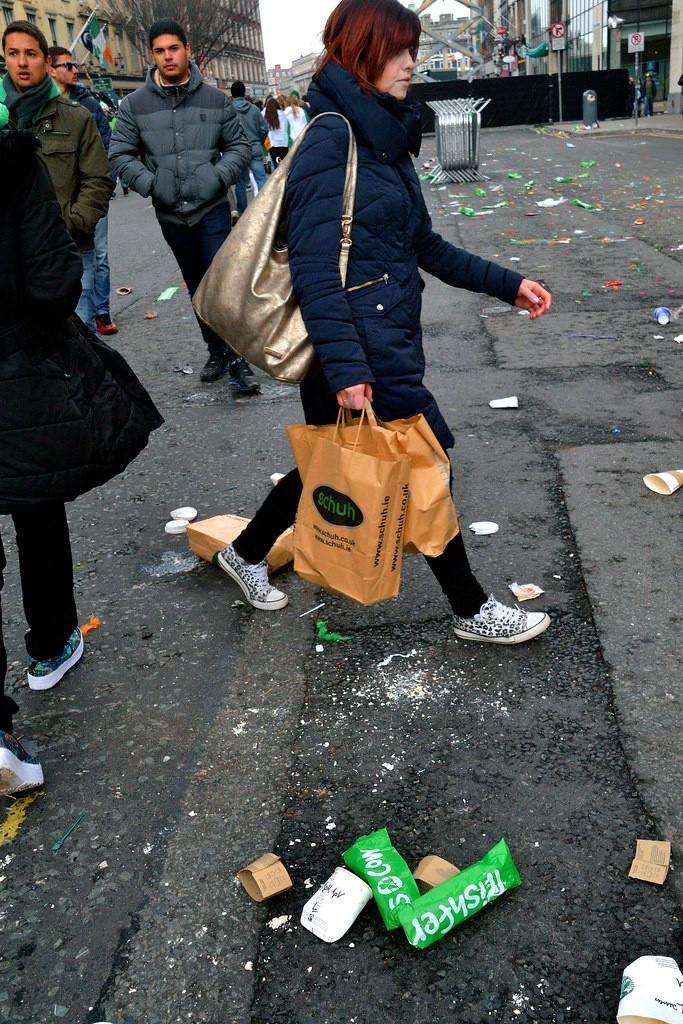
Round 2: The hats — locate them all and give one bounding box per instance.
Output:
[289,91,299,97]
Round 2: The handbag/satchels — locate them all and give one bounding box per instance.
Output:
[191,111,357,384]
[185,397,461,607]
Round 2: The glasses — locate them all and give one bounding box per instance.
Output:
[54,62,78,71]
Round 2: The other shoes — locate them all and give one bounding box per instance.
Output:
[110,192,116,199]
[231,210,239,226]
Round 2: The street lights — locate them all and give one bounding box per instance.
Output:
[114,53,125,98]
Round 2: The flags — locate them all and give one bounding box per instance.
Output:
[77,12,113,67]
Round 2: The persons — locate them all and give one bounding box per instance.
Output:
[0,103,165,795]
[629,77,642,118]
[0,20,311,392]
[217,0,552,645]
[678,73,683,117]
[642,72,656,119]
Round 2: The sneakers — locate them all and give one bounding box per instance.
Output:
[227,356,261,393]
[217,542,288,610]
[96,313,119,335]
[28,627,84,690]
[0,730,44,797]
[201,352,227,381]
[453,593,550,644]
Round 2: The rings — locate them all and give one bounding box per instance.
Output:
[539,298,542,300]
[343,400,348,404]
